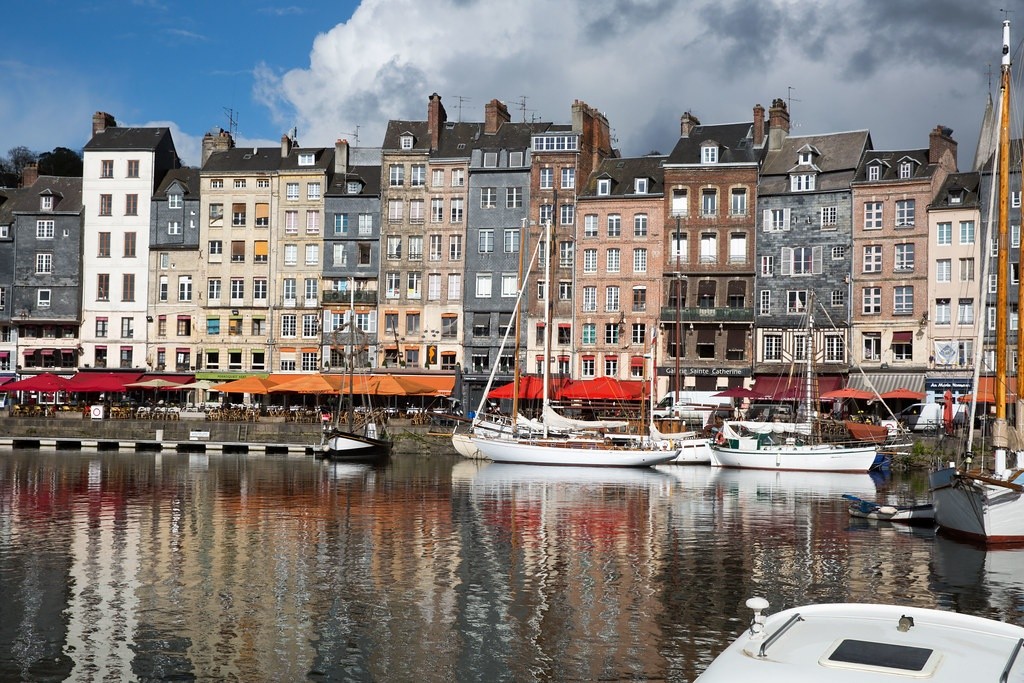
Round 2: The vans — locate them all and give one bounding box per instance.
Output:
[653,390,735,422]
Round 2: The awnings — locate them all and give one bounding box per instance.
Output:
[751,376,844,403]
[22,349,35,355]
[846,375,925,400]
[71,372,143,381]
[0,352,10,360]
[41,348,55,355]
[267,374,456,396]
[963,376,1018,403]
[61,349,73,353]
[891,332,912,344]
[137,375,196,391]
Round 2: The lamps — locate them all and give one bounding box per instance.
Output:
[232,310,239,315]
[146,316,153,322]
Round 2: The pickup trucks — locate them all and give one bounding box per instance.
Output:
[888,404,968,436]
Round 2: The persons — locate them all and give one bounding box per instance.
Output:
[224,401,231,409]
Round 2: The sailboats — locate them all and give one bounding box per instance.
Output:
[709,288,917,474]
[323,276,393,456]
[705,467,876,509]
[453,221,715,467]
[923,10,1024,545]
[450,460,692,598]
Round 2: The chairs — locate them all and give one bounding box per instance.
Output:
[13,402,432,426]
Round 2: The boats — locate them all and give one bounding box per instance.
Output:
[847,503,937,524]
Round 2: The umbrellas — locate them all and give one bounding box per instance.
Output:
[876,388,928,424]
[0,373,97,408]
[957,392,1017,422]
[121,373,437,416]
[821,388,875,412]
[488,375,563,418]
[709,387,765,421]
[556,375,653,415]
[770,387,821,404]
[944,390,953,435]
